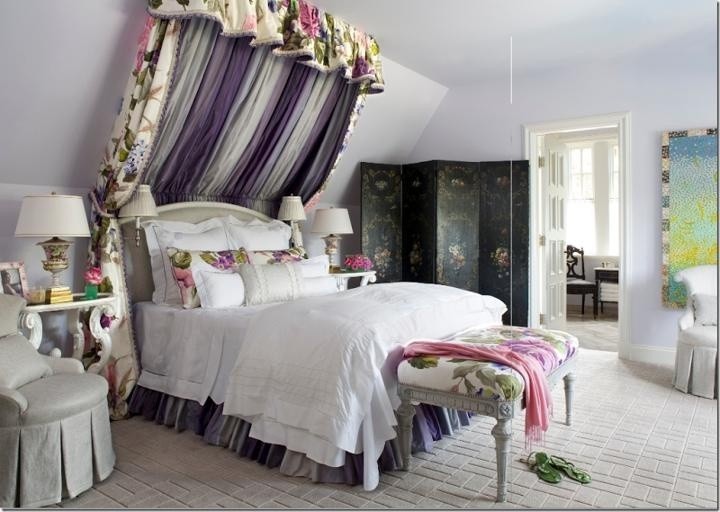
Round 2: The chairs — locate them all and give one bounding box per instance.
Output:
[1,287,114,510]
[671,262,718,401]
[563,243,597,319]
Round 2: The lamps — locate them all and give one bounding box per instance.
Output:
[118,181,155,249]
[309,203,353,272]
[277,193,308,250]
[15,191,94,299]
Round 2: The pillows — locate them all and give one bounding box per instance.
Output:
[691,289,718,328]
[132,215,343,312]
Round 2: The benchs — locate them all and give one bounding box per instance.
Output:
[391,322,582,501]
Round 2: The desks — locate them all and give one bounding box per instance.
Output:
[591,266,618,317]
[327,269,377,292]
[19,290,124,373]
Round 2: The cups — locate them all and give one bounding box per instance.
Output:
[29,290,46,305]
[602,262,610,268]
[83,285,98,299]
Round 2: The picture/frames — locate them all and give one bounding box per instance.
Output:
[1,259,29,301]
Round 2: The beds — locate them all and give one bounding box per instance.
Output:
[104,201,511,483]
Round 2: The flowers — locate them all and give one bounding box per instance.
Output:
[342,251,372,272]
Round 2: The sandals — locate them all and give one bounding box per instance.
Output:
[549,455,591,483]
[536,452,561,482]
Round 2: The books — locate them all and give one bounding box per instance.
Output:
[45,286,74,304]
